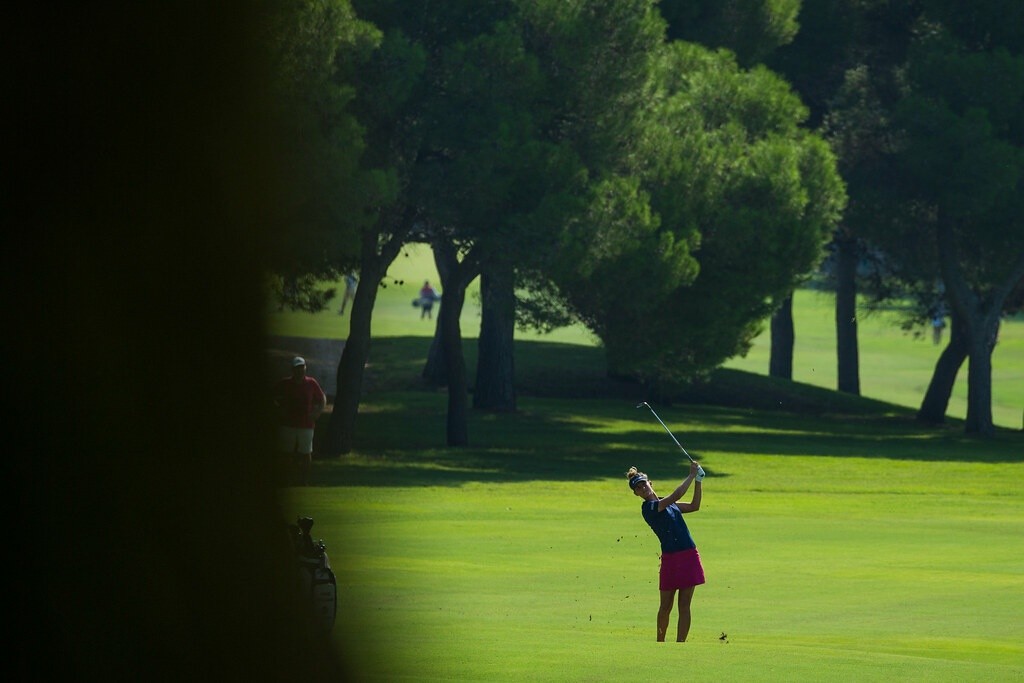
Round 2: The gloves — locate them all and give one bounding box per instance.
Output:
[695,466,705,482]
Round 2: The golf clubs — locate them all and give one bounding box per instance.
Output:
[637,402,704,477]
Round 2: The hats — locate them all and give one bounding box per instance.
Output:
[293,357,305,367]
[630,476,648,486]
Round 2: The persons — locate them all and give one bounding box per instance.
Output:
[419,282,442,319]
[626,460,705,642]
[271,357,326,463]
[336,274,357,315]
